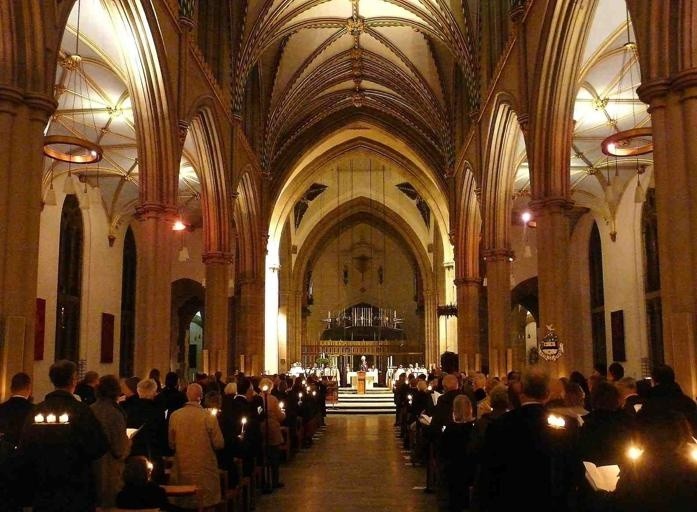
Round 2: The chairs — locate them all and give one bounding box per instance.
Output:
[95,416,320,512]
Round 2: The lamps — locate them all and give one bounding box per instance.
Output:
[602,4,655,202]
[41,0,103,209]
[176,223,195,262]
[524,220,536,258]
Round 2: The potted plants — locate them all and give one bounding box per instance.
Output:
[541,331,558,355]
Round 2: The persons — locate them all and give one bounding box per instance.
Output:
[392,363,697,512]
[361,361,369,372]
[0,358,327,512]
